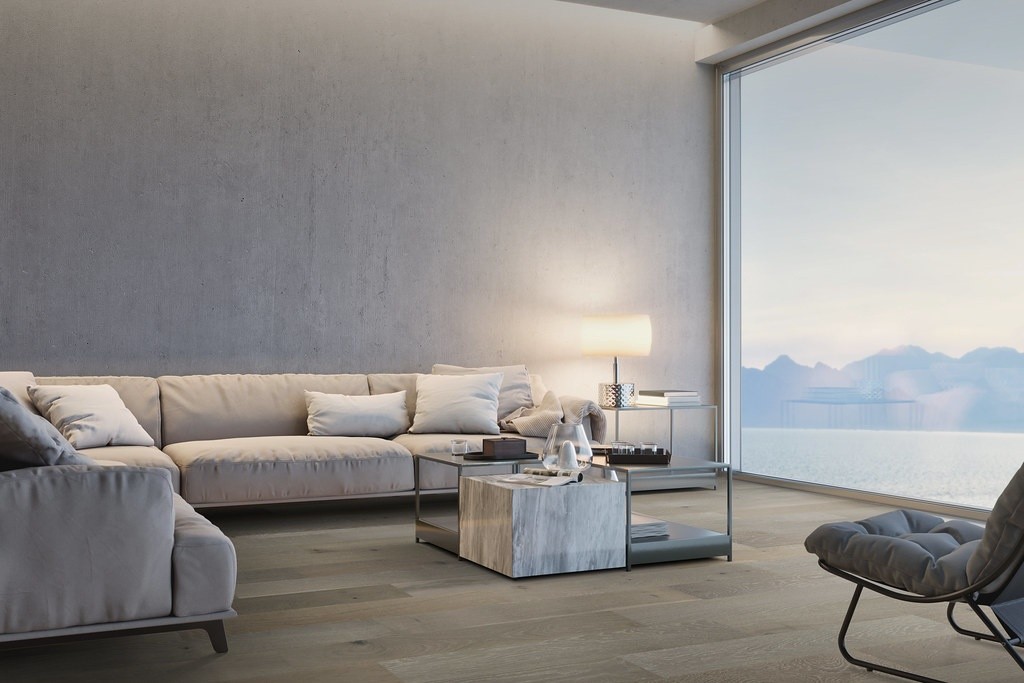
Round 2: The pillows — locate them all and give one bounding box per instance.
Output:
[0,386,97,465]
[407,373,502,435]
[302,388,410,437]
[26,385,155,450]
[431,362,530,421]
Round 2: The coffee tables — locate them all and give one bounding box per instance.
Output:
[413,453,540,560]
[578,454,733,571]
[458,473,628,578]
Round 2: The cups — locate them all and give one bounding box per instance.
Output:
[610,441,658,455]
[451,439,468,456]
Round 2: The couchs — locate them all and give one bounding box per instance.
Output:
[0,374,606,653]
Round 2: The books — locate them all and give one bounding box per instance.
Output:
[497,468,584,487]
[637,390,702,406]
[631,513,670,538]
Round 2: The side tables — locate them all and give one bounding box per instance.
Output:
[602,404,717,491]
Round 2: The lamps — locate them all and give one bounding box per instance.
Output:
[577,313,652,408]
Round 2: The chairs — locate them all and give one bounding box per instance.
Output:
[803,462,1024,683]
